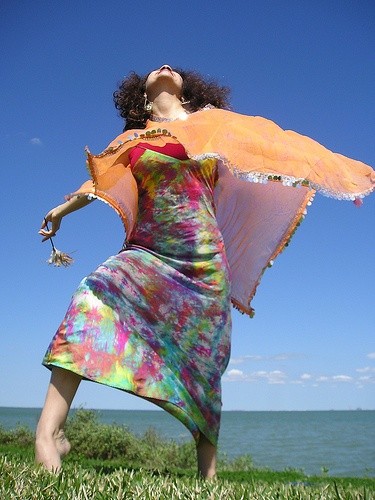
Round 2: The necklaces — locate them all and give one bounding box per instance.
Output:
[148,114,171,122]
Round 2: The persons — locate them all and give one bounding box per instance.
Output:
[33,63,374,489]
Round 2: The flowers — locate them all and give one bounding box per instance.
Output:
[43,217,76,269]
